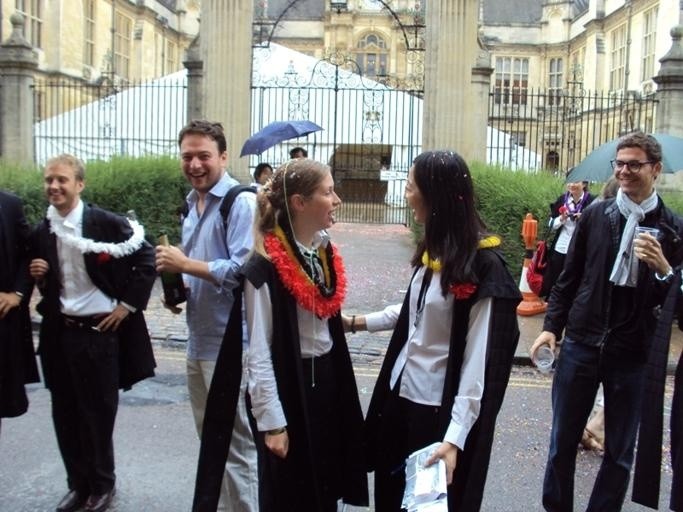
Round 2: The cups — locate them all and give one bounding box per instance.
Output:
[533,348,554,375]
[634,226,659,238]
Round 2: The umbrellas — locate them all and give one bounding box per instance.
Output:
[238,120,325,159]
[561,116,683,183]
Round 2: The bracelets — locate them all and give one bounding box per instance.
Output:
[266,429,284,434]
[16,291,22,296]
[351,315,356,334]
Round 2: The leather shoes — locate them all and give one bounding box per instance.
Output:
[85,485,116,510]
[56,487,85,512]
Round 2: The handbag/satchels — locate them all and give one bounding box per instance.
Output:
[527,240,553,297]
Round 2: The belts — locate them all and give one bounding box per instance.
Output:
[61,315,105,326]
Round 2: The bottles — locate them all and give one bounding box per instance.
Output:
[158,231,186,306]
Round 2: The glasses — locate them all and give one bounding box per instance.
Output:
[610,159,654,173]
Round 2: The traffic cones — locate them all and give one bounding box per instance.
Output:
[515,249,545,316]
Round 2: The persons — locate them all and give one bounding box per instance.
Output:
[538,170,593,342]
[250,163,274,192]
[155,119,259,512]
[189,156,372,512]
[290,147,306,161]
[630,261,683,512]
[0,189,41,420]
[532,132,683,512]
[339,151,524,512]
[23,153,157,512]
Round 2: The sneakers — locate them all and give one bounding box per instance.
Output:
[578,428,604,451]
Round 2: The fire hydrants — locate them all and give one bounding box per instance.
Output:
[521,214,538,246]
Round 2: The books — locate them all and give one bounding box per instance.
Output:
[402,442,448,512]
